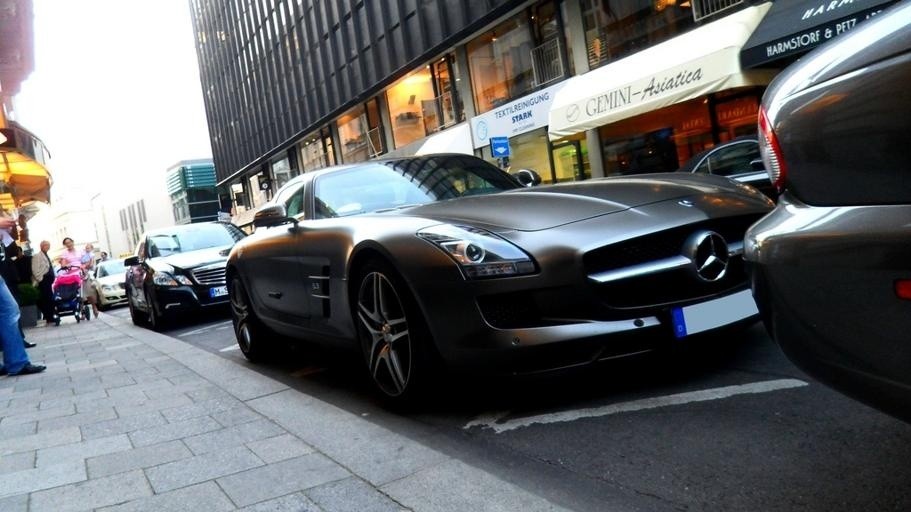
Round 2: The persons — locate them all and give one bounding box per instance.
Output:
[18,319,37,348]
[52,237,113,318]
[0,203,47,377]
[32,241,61,323]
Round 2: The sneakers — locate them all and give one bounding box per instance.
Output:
[80,313,85,320]
[94,311,98,318]
[0,363,46,376]
[43,316,56,322]
[22,339,36,348]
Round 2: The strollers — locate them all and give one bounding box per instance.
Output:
[51,266,90,326]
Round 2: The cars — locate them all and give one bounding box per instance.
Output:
[89,221,249,330]
